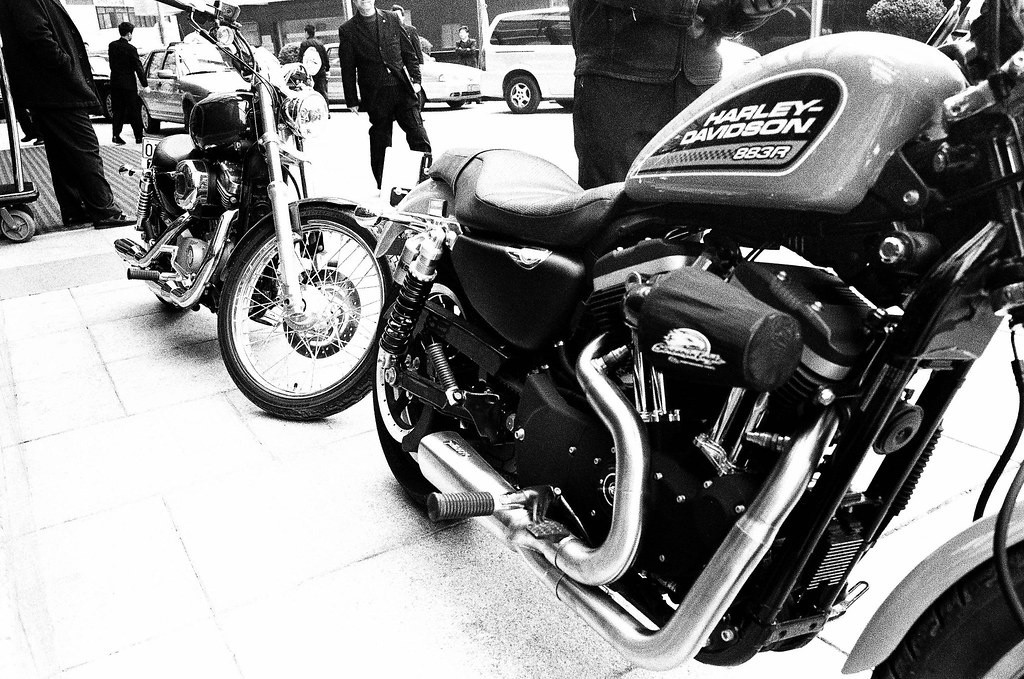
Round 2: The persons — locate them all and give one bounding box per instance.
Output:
[108,22,148,145]
[298,24,331,119]
[0,0,138,228]
[338,0,431,189]
[390,4,425,122]
[455,26,483,104]
[567,0,791,190]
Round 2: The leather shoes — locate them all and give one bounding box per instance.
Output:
[21,136,37,142]
[136,136,145,143]
[93,210,137,229]
[62,215,93,227]
[467,101,471,104]
[34,140,45,145]
[476,102,482,104]
[112,136,126,144]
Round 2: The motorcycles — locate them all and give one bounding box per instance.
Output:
[112,0,403,422]
[350,0,1023,679]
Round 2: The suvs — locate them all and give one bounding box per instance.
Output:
[482,7,764,116]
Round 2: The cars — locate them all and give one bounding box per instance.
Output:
[83,32,482,136]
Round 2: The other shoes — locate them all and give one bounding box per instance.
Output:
[329,114,331,119]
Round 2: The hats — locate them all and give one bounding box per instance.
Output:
[304,25,315,33]
